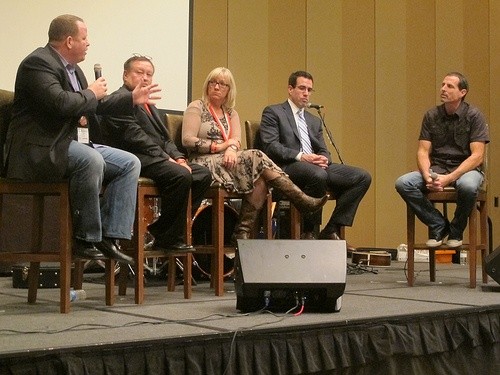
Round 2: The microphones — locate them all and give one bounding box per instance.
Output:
[307,103,324,108]
[95,64,104,103]
[431,173,437,180]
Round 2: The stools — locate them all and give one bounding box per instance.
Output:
[406,123,490,290]
[0,89,347,314]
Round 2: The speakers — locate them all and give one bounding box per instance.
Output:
[234,239,347,313]
[484,245,500,285]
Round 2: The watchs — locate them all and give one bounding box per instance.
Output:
[229,145,238,151]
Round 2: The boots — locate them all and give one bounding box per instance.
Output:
[267,172,329,217]
[231,198,261,246]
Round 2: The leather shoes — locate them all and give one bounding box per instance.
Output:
[304,232,317,240]
[72,238,104,259]
[94,236,133,263]
[318,231,357,251]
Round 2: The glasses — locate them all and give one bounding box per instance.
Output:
[132,52,152,60]
[209,80,229,87]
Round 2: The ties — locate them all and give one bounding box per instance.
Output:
[297,110,312,154]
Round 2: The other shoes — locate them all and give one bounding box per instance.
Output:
[147,225,157,239]
[152,240,195,251]
[425,229,450,247]
[445,228,463,247]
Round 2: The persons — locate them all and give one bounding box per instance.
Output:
[2,15,141,266]
[182,66,330,246]
[394,72,490,248]
[97,53,211,252]
[259,70,372,241]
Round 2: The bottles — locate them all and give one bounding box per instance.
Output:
[459,250,468,265]
[69,289,87,301]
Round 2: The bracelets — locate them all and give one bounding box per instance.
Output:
[178,160,186,165]
[211,142,217,153]
